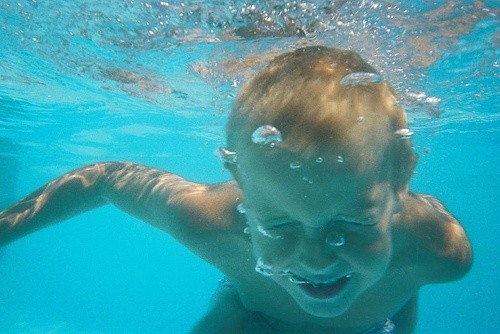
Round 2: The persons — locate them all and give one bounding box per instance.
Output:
[0,41,475,334]
